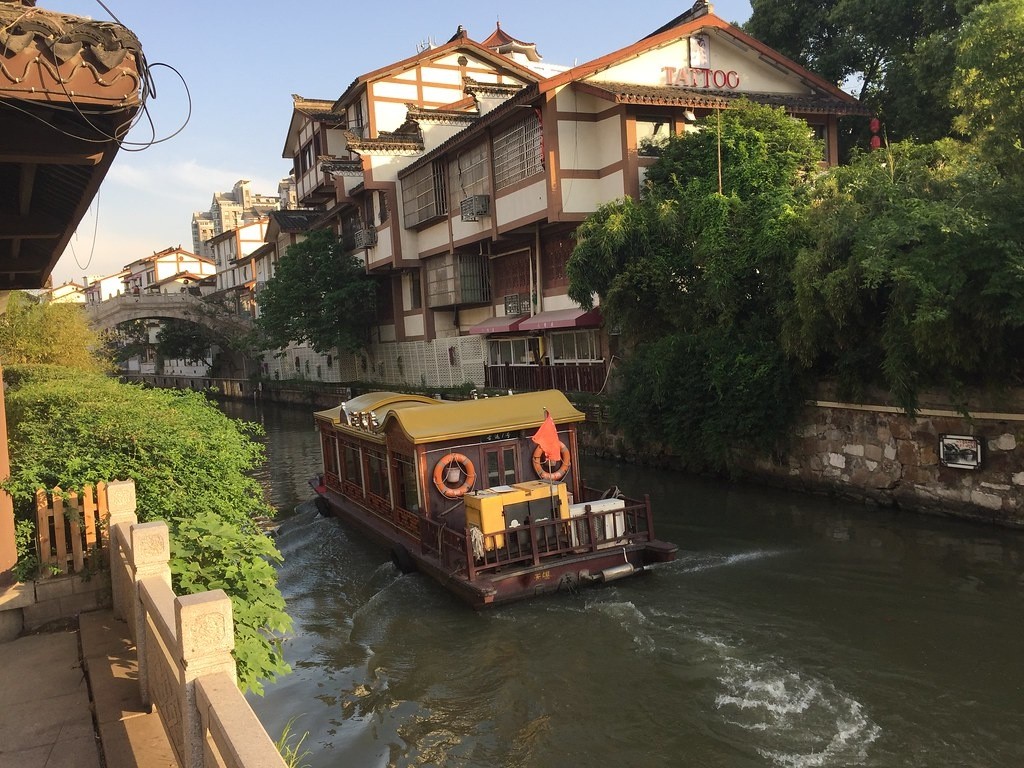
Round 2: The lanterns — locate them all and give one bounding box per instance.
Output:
[870,136,880,149]
[871,118,880,133]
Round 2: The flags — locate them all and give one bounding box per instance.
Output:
[532,410,561,461]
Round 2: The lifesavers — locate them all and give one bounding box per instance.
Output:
[533,440,572,481]
[432,453,475,498]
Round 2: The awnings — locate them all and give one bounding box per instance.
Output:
[518,306,601,331]
[468,312,529,334]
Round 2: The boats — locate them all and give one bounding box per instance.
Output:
[308,389,680,611]
